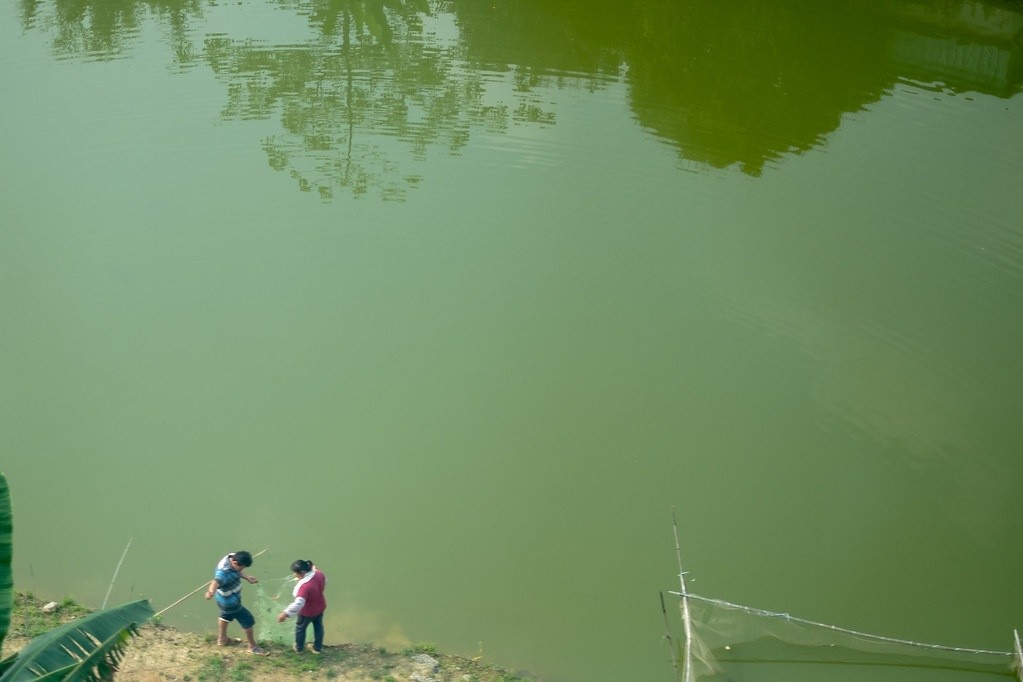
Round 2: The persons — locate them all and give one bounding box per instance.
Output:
[277,560,327,654]
[205,550,271,656]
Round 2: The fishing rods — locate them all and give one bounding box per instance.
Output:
[102,532,135,611]
[134,549,270,629]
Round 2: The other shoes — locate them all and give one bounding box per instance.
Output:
[216,637,242,646]
[306,642,321,655]
[292,643,304,654]
[245,645,271,656]
